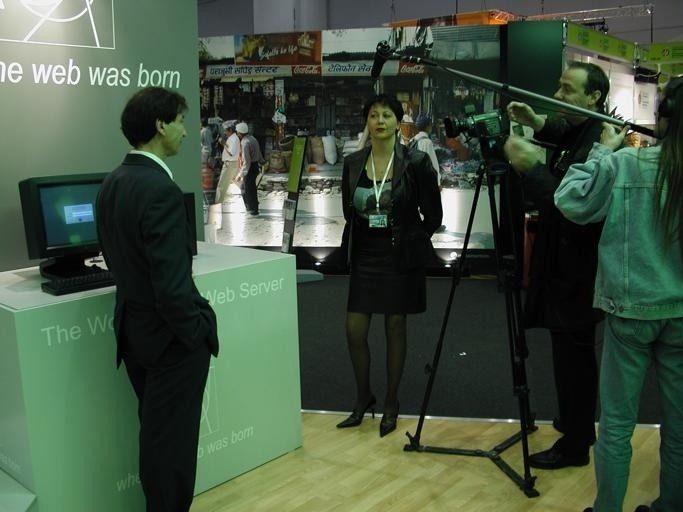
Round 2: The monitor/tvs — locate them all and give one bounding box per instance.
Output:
[17,171,112,275]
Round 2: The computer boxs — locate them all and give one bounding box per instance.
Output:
[180,190,198,257]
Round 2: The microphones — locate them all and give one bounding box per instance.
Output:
[371,40,392,79]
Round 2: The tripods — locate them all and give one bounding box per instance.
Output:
[403,174,541,496]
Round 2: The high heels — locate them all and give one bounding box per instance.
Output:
[379,393,400,438]
[335,392,376,428]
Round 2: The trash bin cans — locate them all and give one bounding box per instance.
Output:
[522,211,539,288]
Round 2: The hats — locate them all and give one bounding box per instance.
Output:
[235,122,249,134]
[221,119,237,129]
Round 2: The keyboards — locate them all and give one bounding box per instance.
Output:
[40,271,114,295]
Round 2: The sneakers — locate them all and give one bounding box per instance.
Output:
[246,213,260,218]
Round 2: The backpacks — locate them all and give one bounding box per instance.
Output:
[407,135,429,151]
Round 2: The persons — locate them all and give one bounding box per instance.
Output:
[506,61,622,469]
[213,119,239,203]
[406,115,446,232]
[233,120,264,216]
[91,87,221,510]
[552,75,680,511]
[336,93,443,438]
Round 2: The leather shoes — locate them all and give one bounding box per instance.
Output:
[553,416,597,446]
[528,435,590,470]
